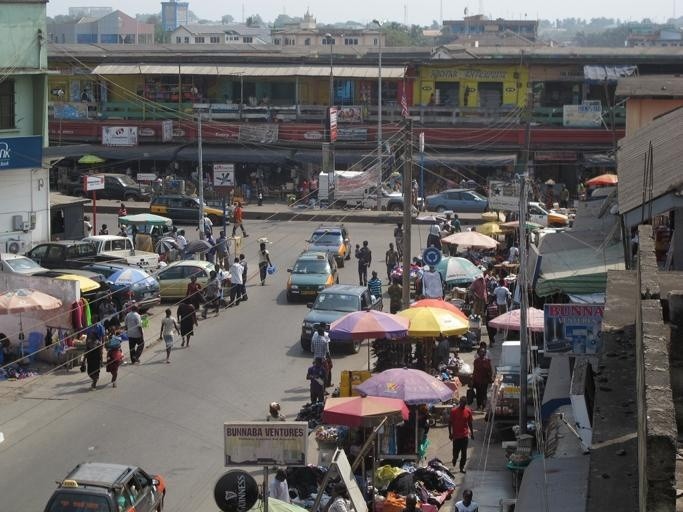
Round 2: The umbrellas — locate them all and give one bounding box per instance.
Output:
[393,298,545,338]
[77,154,104,166]
[438,230,500,249]
[586,171,616,186]
[0,288,63,366]
[49,272,101,294]
[478,211,542,235]
[321,365,454,426]
[328,309,408,371]
[102,264,160,304]
[113,211,172,234]
[418,255,484,284]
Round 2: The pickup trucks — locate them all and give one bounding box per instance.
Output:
[301,283,381,354]
[81,234,158,269]
[22,241,122,278]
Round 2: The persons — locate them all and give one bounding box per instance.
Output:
[193,89,272,103]
[79,202,274,391]
[53,210,65,233]
[266,466,350,512]
[453,490,479,512]
[426,91,436,105]
[80,88,89,103]
[448,396,474,474]
[442,172,571,210]
[401,494,421,512]
[266,213,520,421]
[254,173,320,206]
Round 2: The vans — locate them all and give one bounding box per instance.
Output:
[316,170,403,209]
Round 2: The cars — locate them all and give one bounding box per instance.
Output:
[423,187,490,211]
[153,259,230,299]
[286,248,338,299]
[526,200,568,225]
[75,172,153,201]
[38,458,164,511]
[307,225,352,263]
[149,195,230,224]
[0,252,100,297]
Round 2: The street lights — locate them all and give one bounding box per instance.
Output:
[321,28,338,167]
[370,17,387,208]
